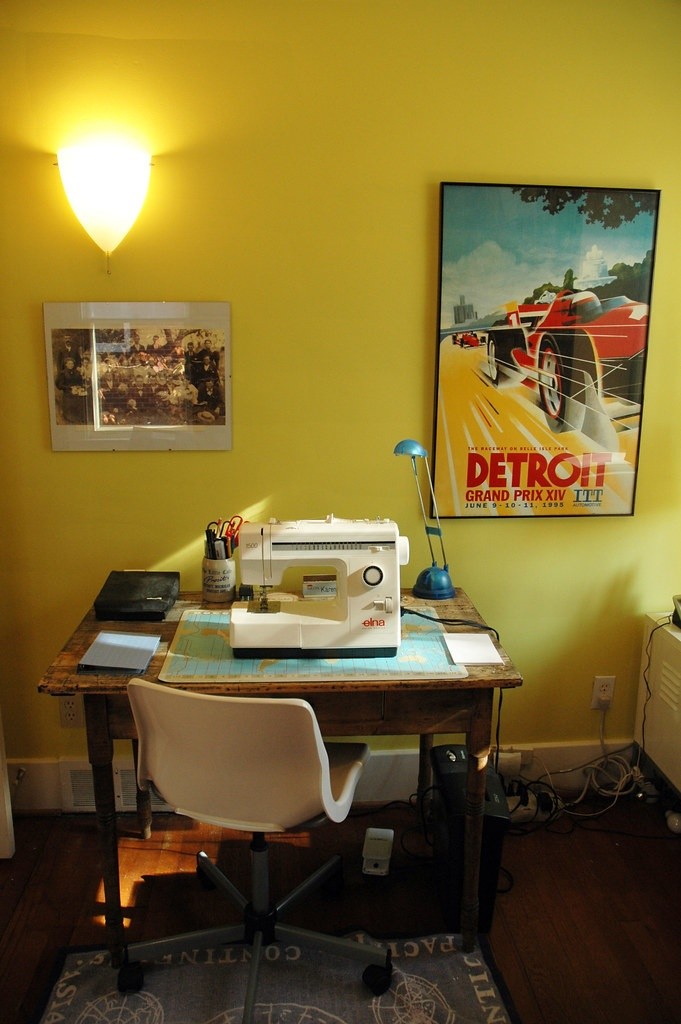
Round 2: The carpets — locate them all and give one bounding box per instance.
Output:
[28,924,525,1024]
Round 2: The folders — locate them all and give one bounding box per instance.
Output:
[76,629,162,674]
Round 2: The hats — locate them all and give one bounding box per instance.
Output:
[197,411,215,423]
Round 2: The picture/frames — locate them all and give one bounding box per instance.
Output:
[427,181,662,519]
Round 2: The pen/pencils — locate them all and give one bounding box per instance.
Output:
[204,518,236,560]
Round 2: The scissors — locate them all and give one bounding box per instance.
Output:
[207,516,250,544]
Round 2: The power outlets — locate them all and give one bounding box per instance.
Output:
[591,676,616,709]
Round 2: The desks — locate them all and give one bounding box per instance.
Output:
[38,586,522,936]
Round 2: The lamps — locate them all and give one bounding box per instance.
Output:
[394,439,455,600]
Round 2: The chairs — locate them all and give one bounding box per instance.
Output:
[118,679,393,1023]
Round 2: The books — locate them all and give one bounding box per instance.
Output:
[77,630,162,675]
[444,632,505,666]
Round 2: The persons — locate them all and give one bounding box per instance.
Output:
[57,334,224,425]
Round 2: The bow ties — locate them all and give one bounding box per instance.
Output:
[207,387,213,393]
[136,388,144,392]
